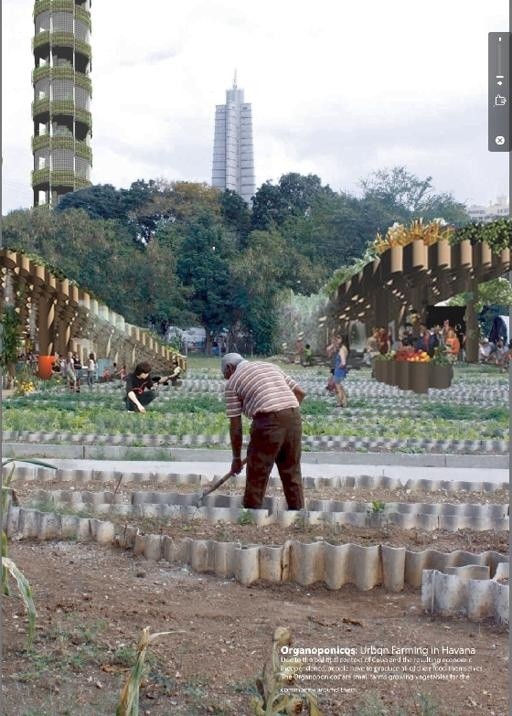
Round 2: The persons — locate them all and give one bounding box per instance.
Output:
[51,350,182,414]
[221,352,306,510]
[304,309,512,408]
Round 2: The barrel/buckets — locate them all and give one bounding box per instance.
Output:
[375,359,453,393]
[39,356,57,379]
